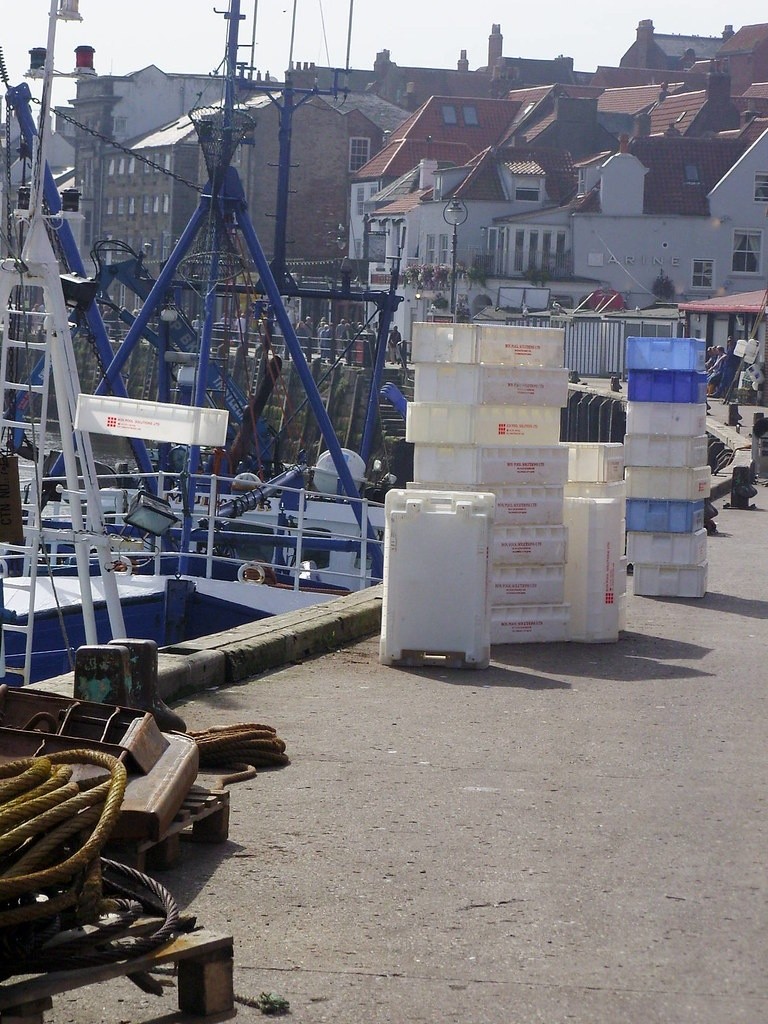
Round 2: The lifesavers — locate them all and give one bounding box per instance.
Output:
[111,555,132,576]
[238,563,265,584]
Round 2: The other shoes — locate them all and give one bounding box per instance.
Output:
[706,392,720,398]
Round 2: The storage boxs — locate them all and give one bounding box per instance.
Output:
[71,392,230,447]
[378,321,711,670]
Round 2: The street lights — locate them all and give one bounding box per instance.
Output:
[354,276,373,326]
[324,232,348,342]
[445,189,468,316]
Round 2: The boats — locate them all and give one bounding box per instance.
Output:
[0,1,401,690]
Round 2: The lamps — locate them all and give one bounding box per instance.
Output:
[121,491,180,537]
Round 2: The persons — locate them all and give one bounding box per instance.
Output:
[294,316,314,363]
[30,300,140,343]
[389,326,402,365]
[317,316,335,364]
[705,335,740,397]
[337,318,376,366]
[173,309,273,359]
